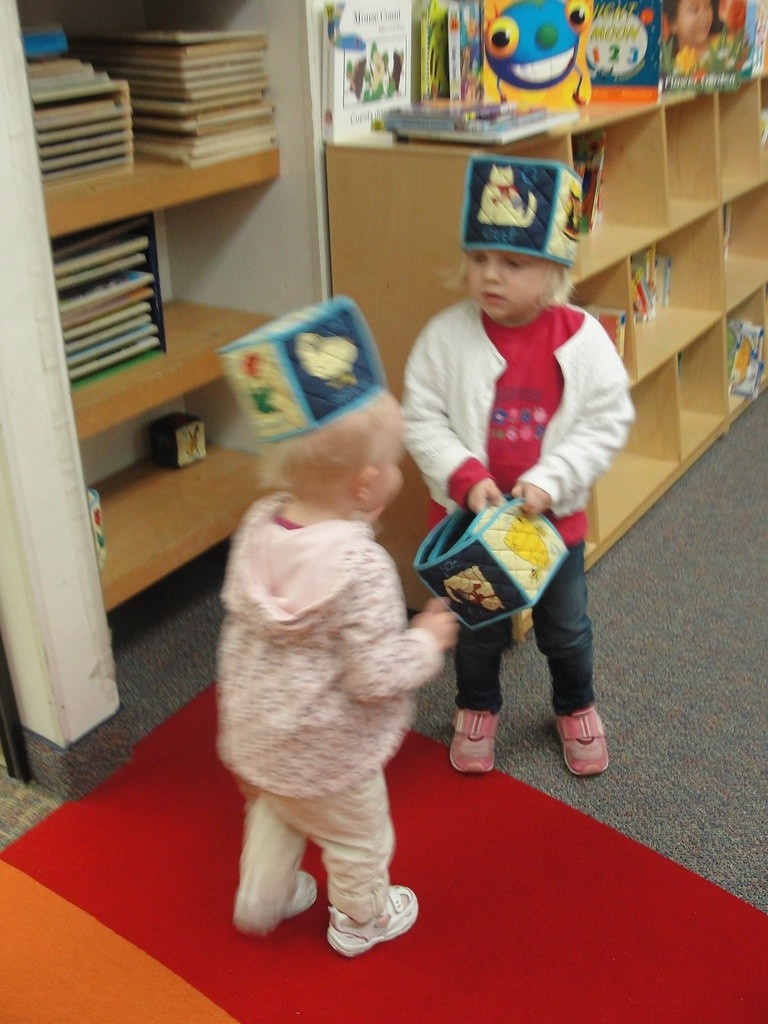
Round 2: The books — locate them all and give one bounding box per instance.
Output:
[383,0,768,146]
[575,131,766,399]
[322,0,412,143]
[22,27,280,389]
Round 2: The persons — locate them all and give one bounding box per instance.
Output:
[402,149,635,780]
[213,298,459,957]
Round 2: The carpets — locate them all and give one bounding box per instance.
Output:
[0,681,768,1024]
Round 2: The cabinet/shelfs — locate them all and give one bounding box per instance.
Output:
[40,150,280,614]
[324,75,768,616]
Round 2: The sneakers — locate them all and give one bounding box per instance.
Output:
[275,870,318,916]
[450,706,499,770]
[557,705,608,774]
[326,884,418,957]
